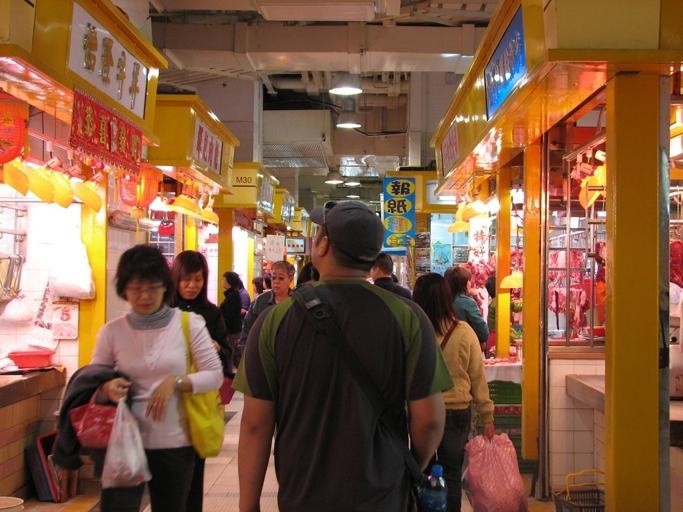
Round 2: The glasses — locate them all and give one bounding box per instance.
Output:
[322,199,338,235]
[271,275,285,281]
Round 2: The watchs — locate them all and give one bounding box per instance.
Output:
[171,375,184,393]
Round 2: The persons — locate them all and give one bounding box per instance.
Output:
[219,271,242,374]
[485,275,523,333]
[167,249,227,512]
[90,244,224,512]
[236,200,455,512]
[370,253,413,299]
[238,279,251,321]
[254,272,272,302]
[252,276,264,298]
[406,273,495,512]
[444,266,490,353]
[240,261,296,343]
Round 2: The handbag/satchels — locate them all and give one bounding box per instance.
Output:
[180,312,224,459]
[69,383,117,450]
[219,370,237,404]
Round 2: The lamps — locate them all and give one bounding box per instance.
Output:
[56,174,76,208]
[328,74,363,96]
[29,165,56,203]
[336,97,362,129]
[77,183,102,210]
[579,175,597,209]
[344,177,361,186]
[448,219,470,233]
[324,166,344,185]
[2,156,29,196]
[202,196,220,225]
[500,271,524,289]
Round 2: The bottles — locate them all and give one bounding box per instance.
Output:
[423,465,447,512]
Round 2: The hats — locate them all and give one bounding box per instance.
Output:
[309,201,386,261]
